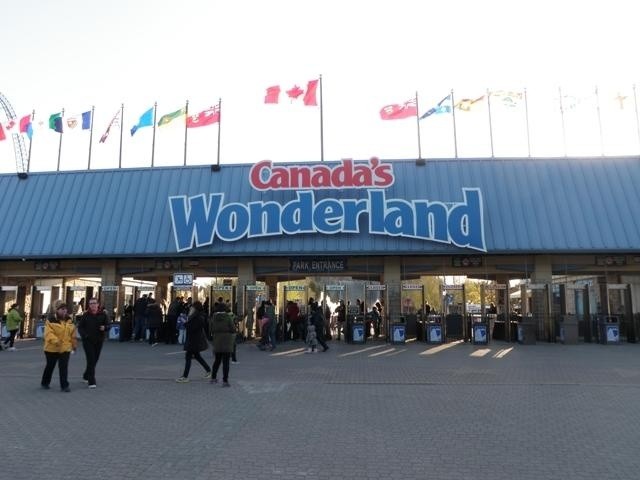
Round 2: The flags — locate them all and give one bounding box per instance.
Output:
[379,97,417,120]
[49,112,63,133]
[457,93,488,111]
[186,104,220,127]
[495,92,525,106]
[7,113,33,139]
[131,107,154,137]
[99,108,122,142]
[159,107,186,127]
[265,78,320,105]
[81,111,91,129]
[421,94,453,121]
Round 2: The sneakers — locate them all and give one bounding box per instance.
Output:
[203,371,212,379]
[175,376,189,383]
[80,378,88,383]
[89,384,96,389]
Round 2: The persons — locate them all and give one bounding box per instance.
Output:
[176,300,212,383]
[416,299,431,323]
[207,302,236,387]
[40,300,77,392]
[485,302,496,314]
[1,304,25,351]
[77,298,111,388]
[74,293,232,347]
[256,297,382,351]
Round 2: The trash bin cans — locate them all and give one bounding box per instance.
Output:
[560,315,579,344]
[473,316,488,344]
[352,316,364,344]
[427,316,442,344]
[392,316,406,344]
[604,316,620,345]
[518,317,537,345]
